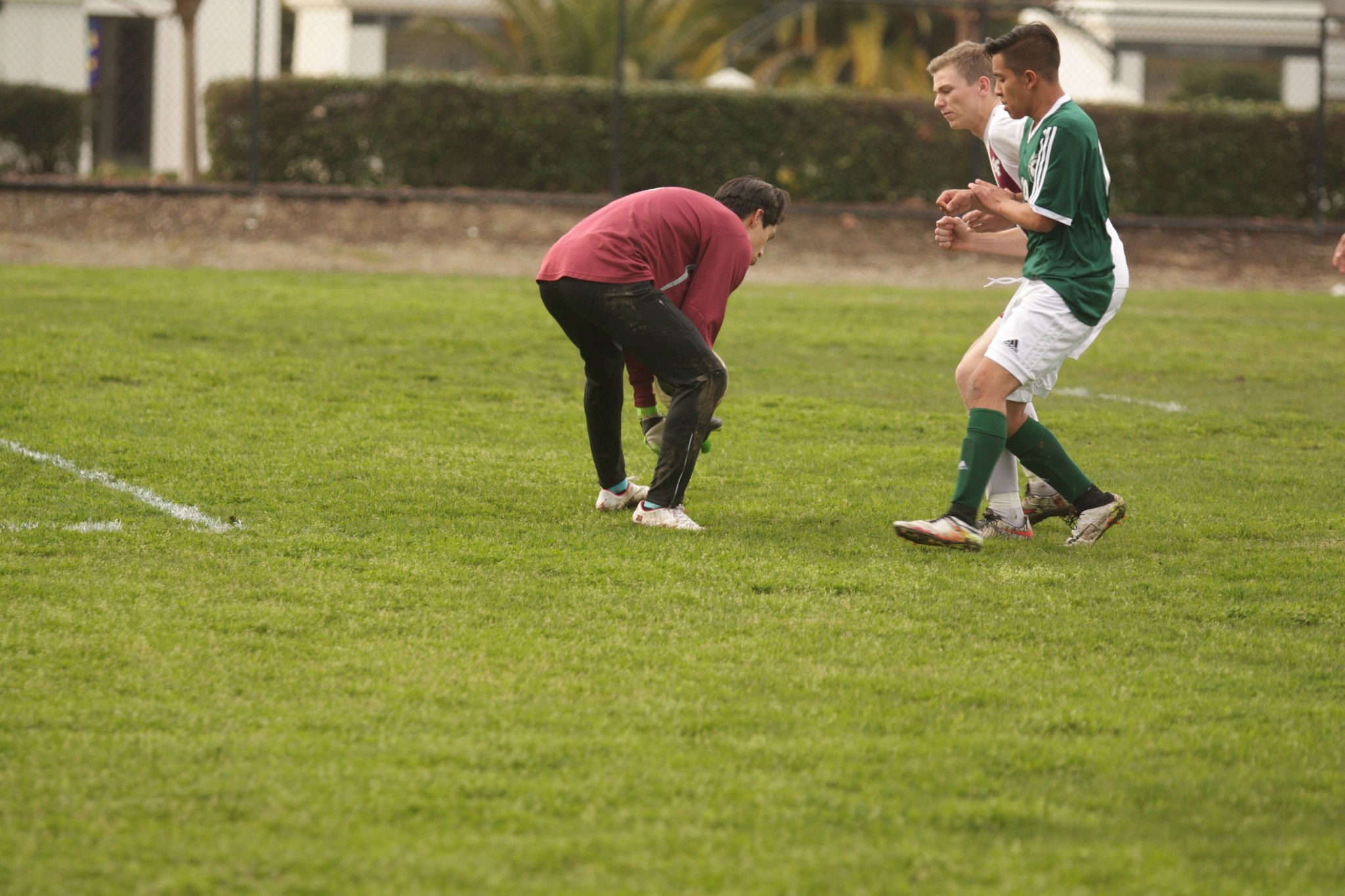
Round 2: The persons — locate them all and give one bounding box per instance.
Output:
[925,40,1131,534]
[893,18,1128,550]
[535,177,792,537]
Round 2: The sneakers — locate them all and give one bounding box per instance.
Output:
[631,500,707,531]
[1021,493,1076,526]
[976,515,1033,540]
[892,515,984,551]
[1063,492,1127,547]
[595,481,650,510]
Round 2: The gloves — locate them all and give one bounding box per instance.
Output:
[640,413,666,455]
[701,417,723,453]
